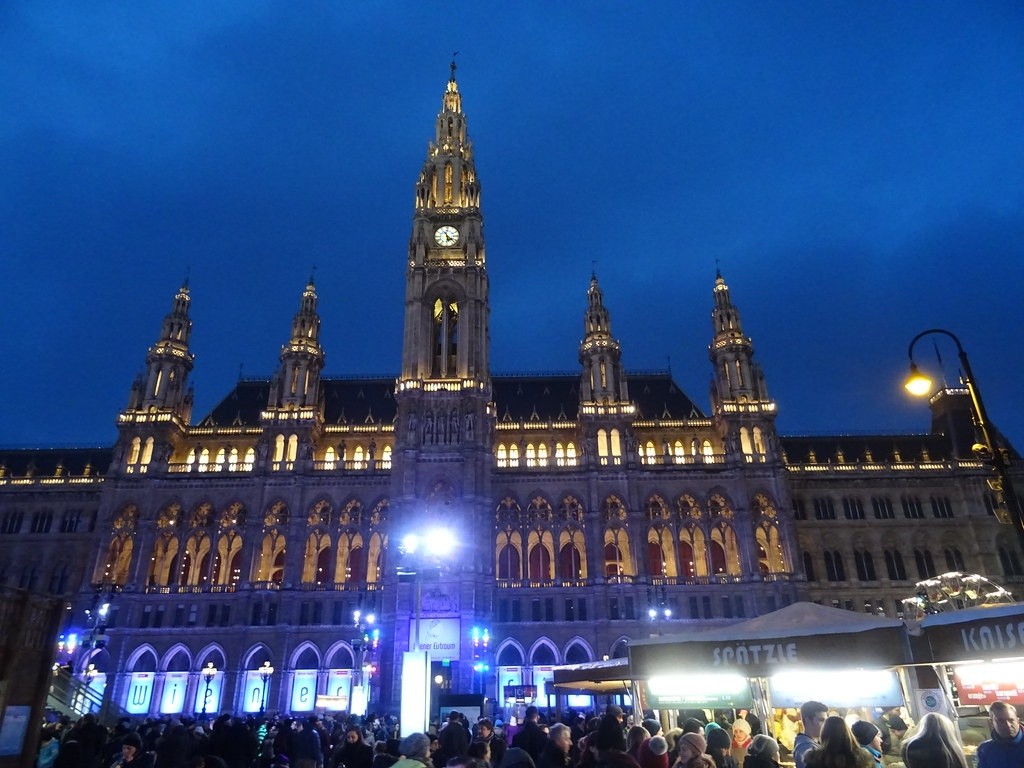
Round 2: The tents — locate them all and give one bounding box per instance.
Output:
[550,655,631,724]
[627,601,919,723]
[922,603,1024,715]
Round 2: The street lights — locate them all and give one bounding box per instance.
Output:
[199,659,217,723]
[903,328,1023,562]
[259,658,274,714]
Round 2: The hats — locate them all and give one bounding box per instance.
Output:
[502,747,535,767]
[851,721,878,745]
[748,734,777,759]
[121,731,143,749]
[398,734,429,757]
[888,713,907,730]
[606,702,750,761]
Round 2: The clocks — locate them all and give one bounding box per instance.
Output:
[433,224,460,249]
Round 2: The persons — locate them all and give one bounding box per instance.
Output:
[799,716,874,768]
[977,702,1024,768]
[794,702,828,768]
[39,706,794,768]
[885,714,909,737]
[900,712,968,768]
[851,719,883,767]
[876,707,906,756]
